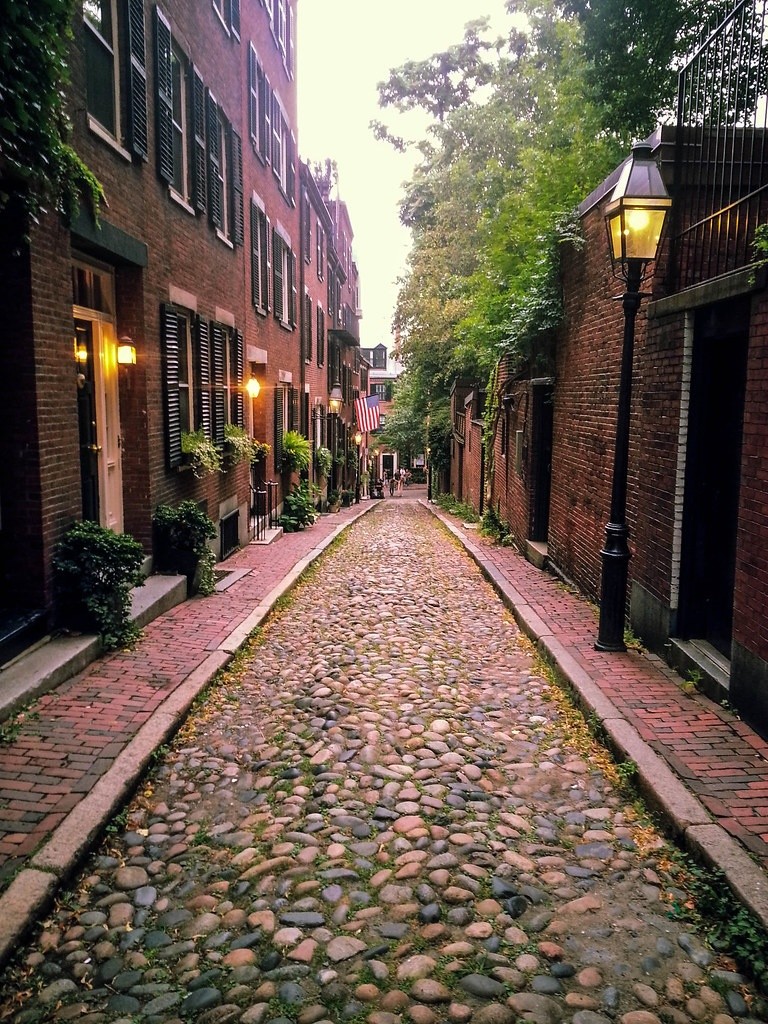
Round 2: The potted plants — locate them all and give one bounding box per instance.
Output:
[156,501,220,584]
[179,430,359,476]
[52,515,148,636]
[327,488,356,513]
[279,478,315,533]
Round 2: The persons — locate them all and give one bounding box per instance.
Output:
[384,467,412,498]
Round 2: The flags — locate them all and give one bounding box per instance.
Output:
[354,393,382,433]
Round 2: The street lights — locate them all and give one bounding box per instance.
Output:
[592,132,674,654]
[327,382,344,501]
[354,435,362,504]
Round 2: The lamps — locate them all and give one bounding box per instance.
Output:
[309,376,344,426]
[349,428,362,447]
[116,334,137,392]
[246,360,260,398]
